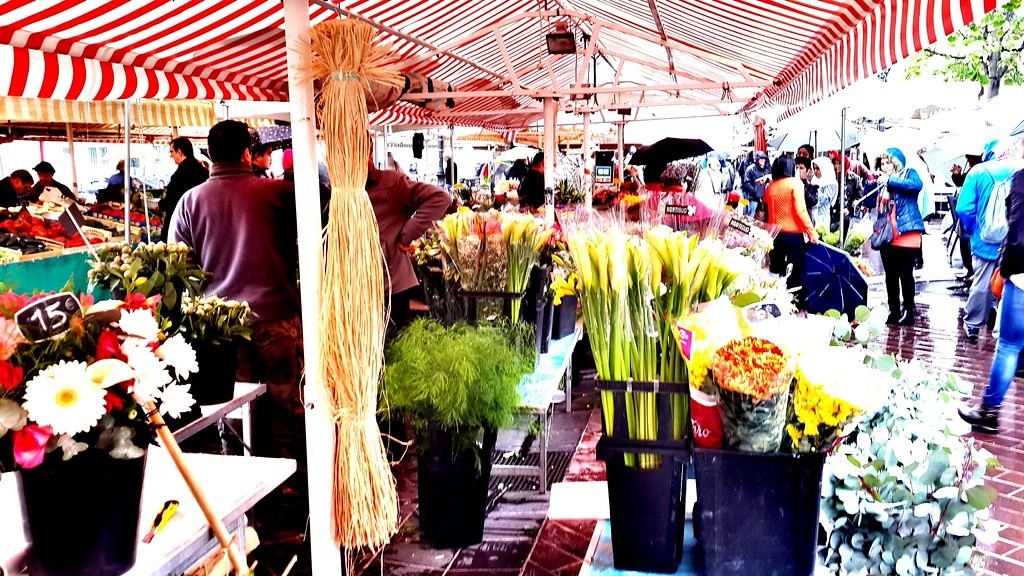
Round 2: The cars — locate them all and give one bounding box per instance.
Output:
[146,165,174,189]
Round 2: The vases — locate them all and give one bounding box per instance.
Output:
[530,299,825,576]
[15,342,240,576]
[421,271,446,324]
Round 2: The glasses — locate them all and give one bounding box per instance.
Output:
[814,167,820,171]
[170,149,174,154]
[798,151,809,156]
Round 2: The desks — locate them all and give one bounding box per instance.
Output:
[490,325,584,494]
[0,210,163,305]
[171,381,267,458]
[18,451,297,576]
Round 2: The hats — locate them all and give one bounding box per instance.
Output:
[831,154,851,176]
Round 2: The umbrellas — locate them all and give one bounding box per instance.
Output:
[247,126,294,151]
[629,135,715,167]
[497,146,541,163]
[766,115,860,155]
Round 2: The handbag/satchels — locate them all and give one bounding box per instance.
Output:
[871,218,894,250]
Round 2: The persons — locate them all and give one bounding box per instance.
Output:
[0,121,1024,434]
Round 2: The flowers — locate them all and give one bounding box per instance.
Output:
[0,237,256,473]
[412,205,870,448]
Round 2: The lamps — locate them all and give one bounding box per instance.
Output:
[546,22,631,116]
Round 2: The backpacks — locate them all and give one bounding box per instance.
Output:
[980,161,1016,245]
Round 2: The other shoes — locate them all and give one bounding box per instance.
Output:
[963,322,980,340]
[898,309,915,326]
[885,310,901,324]
[957,402,1004,431]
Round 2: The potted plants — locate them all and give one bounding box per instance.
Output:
[383,316,539,548]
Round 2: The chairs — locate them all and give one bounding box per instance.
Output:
[945,196,961,263]
